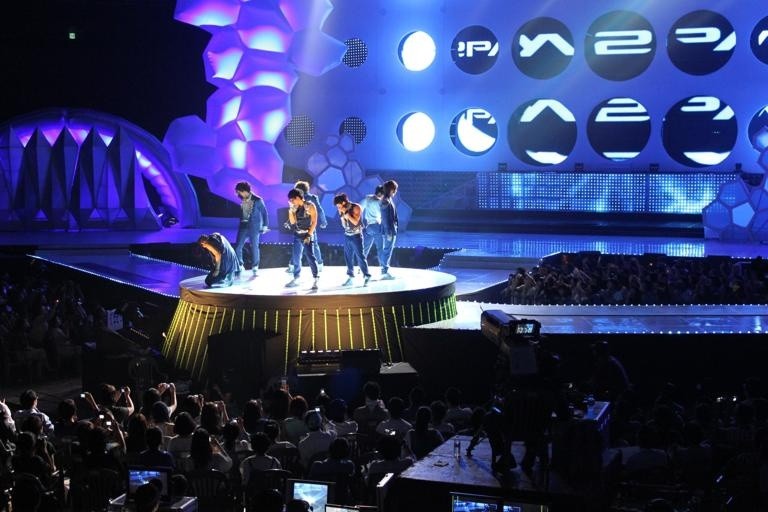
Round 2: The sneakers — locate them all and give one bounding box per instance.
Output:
[238,264,323,277]
[363,277,371,288]
[341,277,353,288]
[380,271,396,281]
[312,278,320,290]
[285,278,300,288]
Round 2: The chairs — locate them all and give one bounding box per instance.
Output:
[0,371,485,510]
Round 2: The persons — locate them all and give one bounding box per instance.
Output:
[356,184,386,276]
[284,189,320,291]
[377,180,400,281]
[233,182,269,280]
[197,231,242,289]
[0,267,147,359]
[333,194,372,288]
[213,232,238,286]
[282,181,328,273]
[500,250,765,307]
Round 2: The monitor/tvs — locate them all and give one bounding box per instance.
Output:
[449,491,551,512]
[126,465,172,504]
[286,478,377,512]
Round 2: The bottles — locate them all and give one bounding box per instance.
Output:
[546,437,553,458]
[454,435,462,458]
